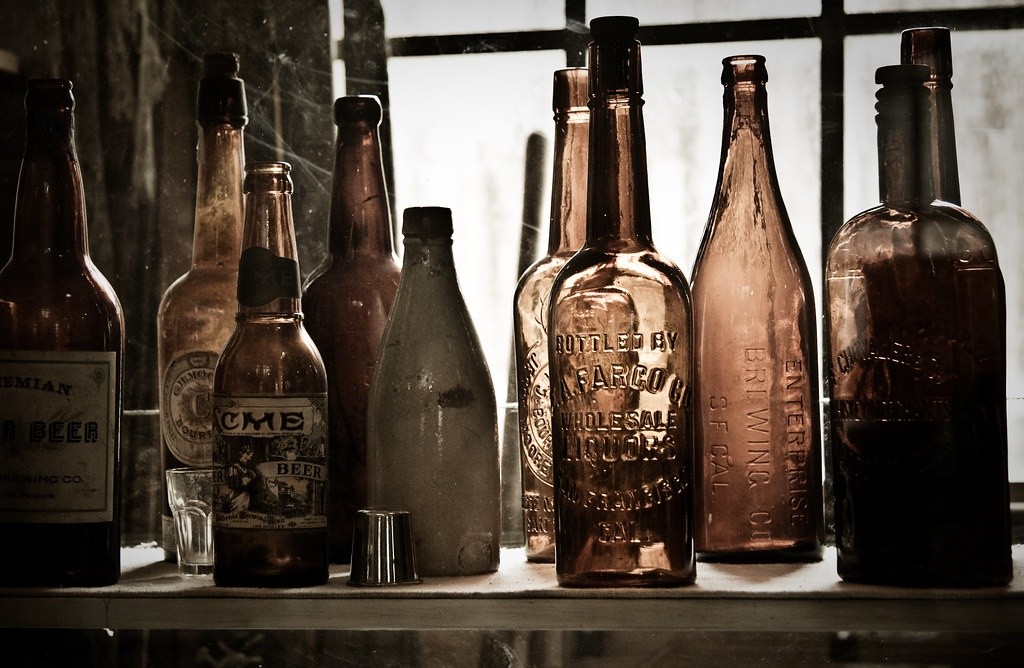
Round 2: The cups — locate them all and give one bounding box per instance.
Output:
[163,466,232,575]
[349,508,421,584]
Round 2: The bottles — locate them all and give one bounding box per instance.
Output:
[0,16,1011,587]
